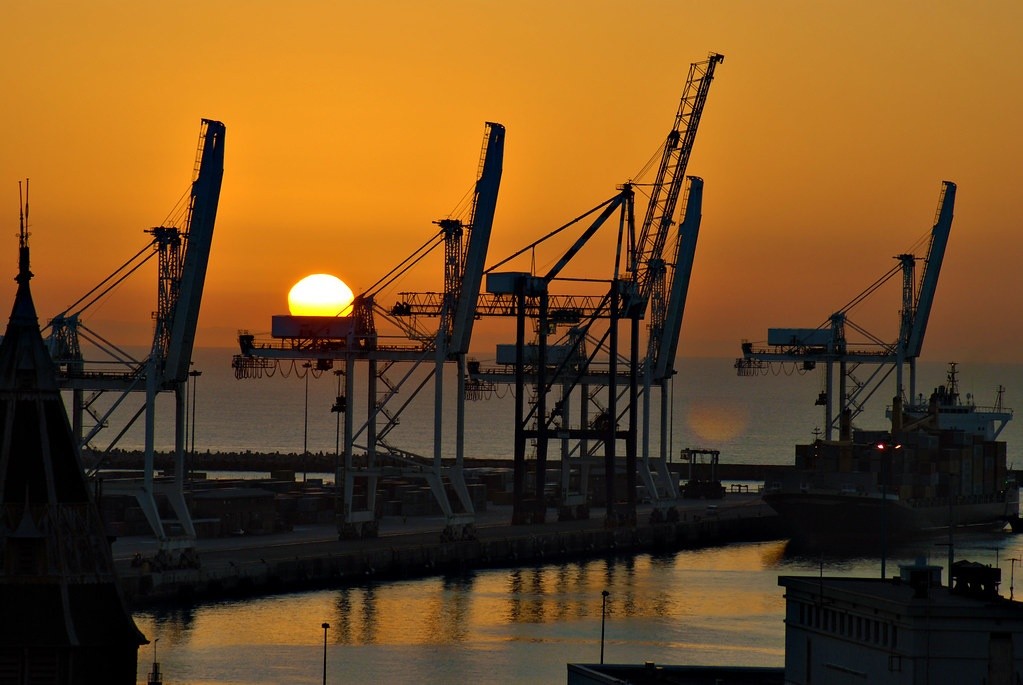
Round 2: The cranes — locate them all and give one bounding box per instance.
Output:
[35,49,722,538]
[733,180,957,451]
